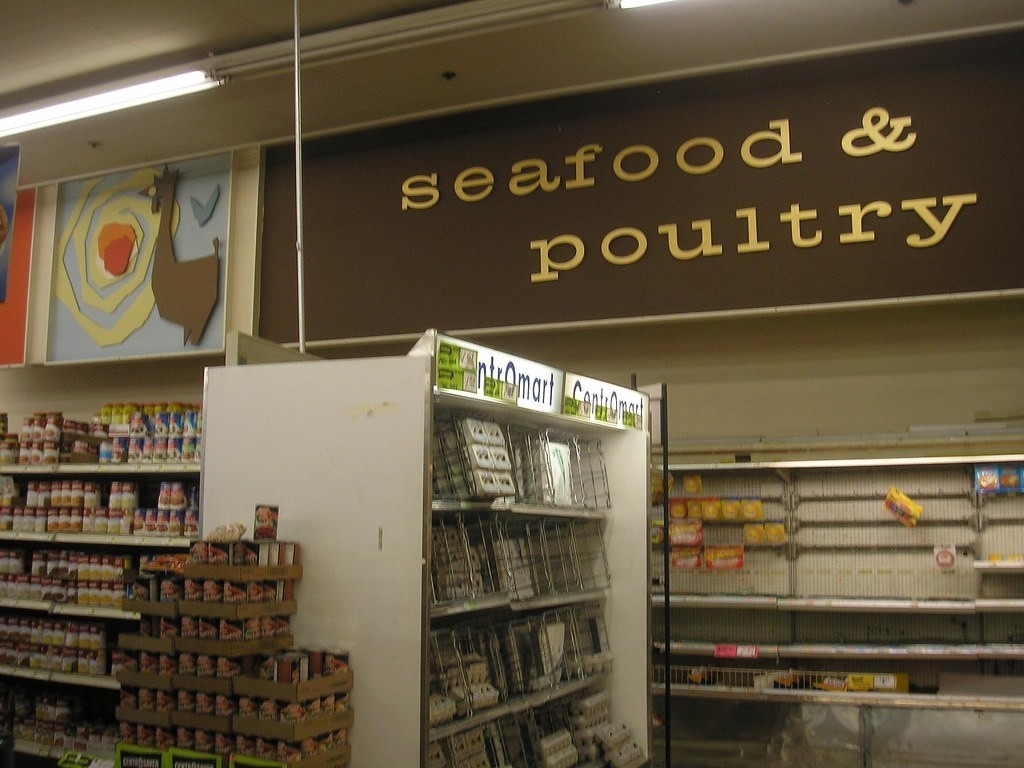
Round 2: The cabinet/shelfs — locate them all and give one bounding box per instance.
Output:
[650,453,1024,711]
[0,461,200,763]
[200,329,654,768]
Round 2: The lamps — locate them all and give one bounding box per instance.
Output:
[0,70,232,138]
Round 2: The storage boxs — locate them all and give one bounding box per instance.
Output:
[61,565,353,768]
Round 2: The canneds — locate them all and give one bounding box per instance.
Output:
[0,402,348,762]
